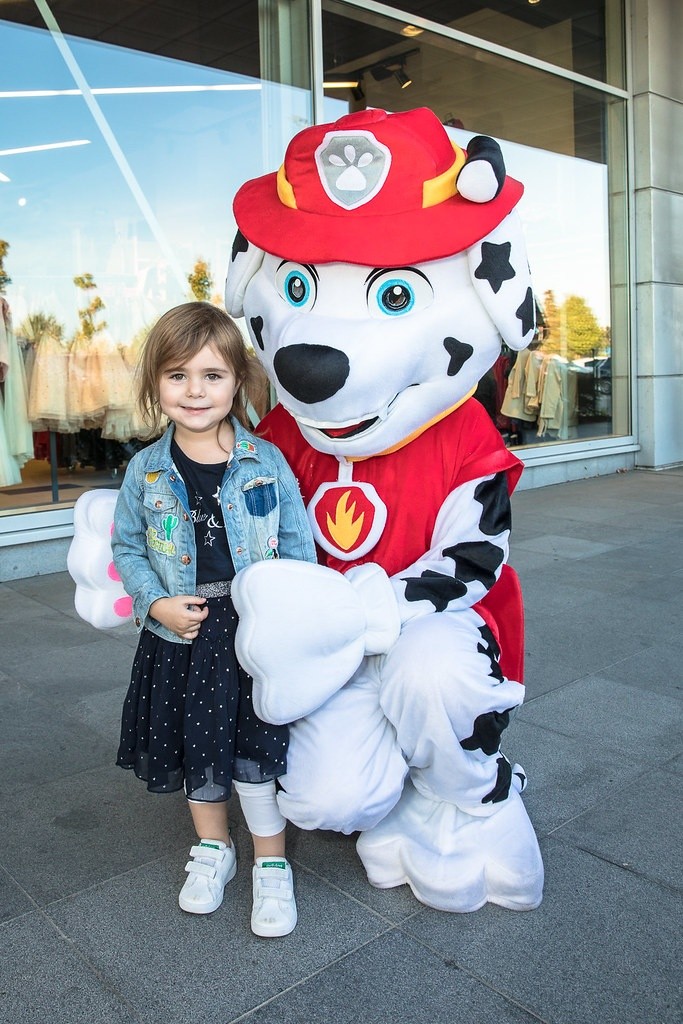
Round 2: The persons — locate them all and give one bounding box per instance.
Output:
[111,303,317,939]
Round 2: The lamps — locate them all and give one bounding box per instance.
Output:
[349,69,365,101]
[383,55,411,90]
[323,74,358,88]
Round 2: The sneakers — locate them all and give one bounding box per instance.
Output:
[251,856,298,938]
[177,837,237,914]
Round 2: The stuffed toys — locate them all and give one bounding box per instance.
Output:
[72,108,544,909]
